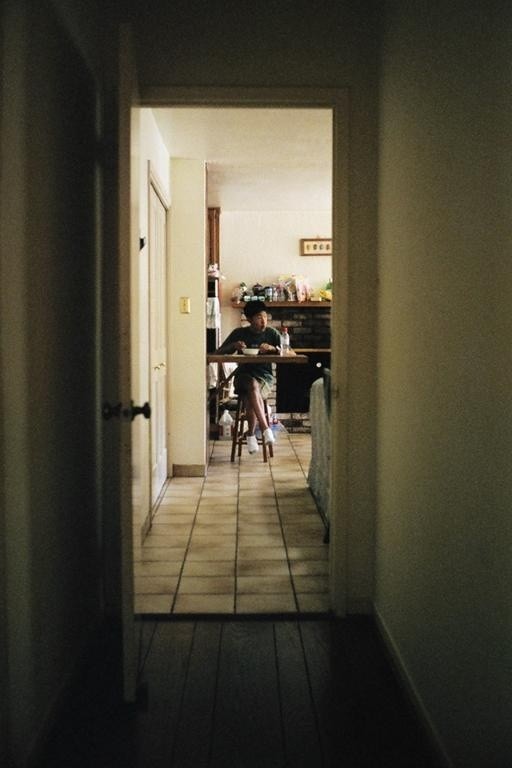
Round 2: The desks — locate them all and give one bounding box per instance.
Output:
[207,354,309,407]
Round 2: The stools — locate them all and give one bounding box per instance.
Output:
[230,391,273,463]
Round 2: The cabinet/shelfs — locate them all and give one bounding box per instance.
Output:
[207,271,221,440]
[231,301,330,353]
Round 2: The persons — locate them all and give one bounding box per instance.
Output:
[217,300,298,455]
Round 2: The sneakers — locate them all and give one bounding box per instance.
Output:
[264,429,275,444]
[246,435,259,454]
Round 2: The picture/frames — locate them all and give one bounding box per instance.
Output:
[301,238,333,256]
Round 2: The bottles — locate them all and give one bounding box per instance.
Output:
[326,278,332,301]
[280,328,290,356]
[245,283,278,302]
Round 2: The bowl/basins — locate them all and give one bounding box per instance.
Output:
[242,349,260,355]
